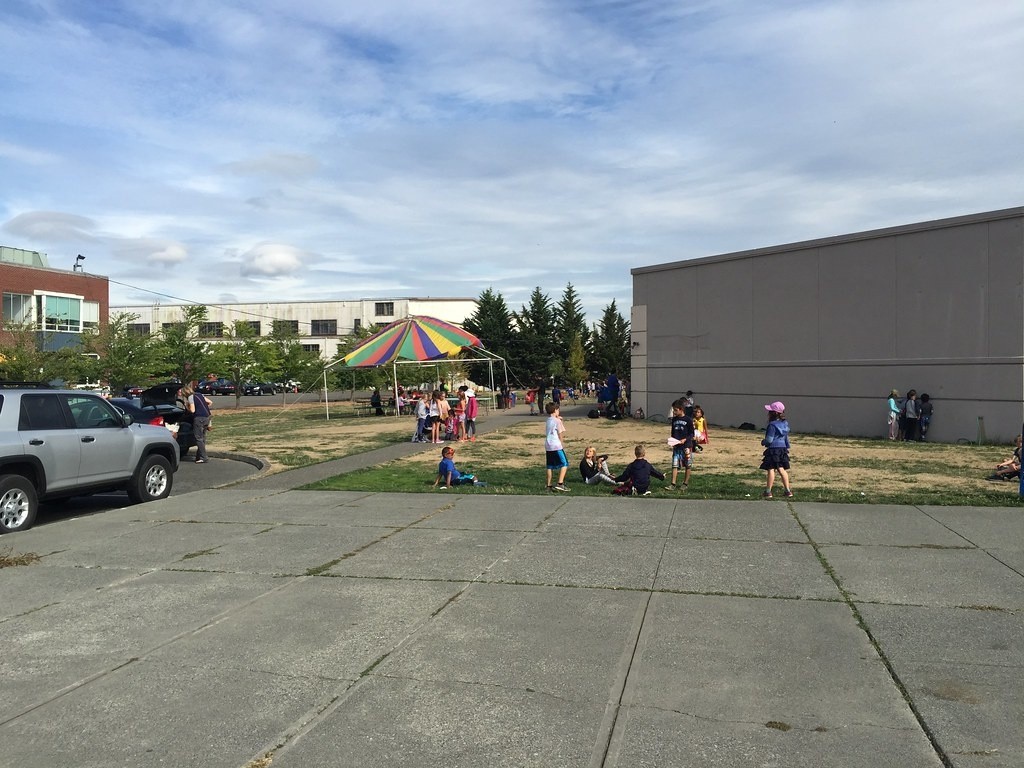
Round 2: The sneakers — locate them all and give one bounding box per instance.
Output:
[666,485,676,490]
[556,484,572,492]
[679,485,688,490]
[761,492,772,498]
[546,486,556,492]
[783,492,794,497]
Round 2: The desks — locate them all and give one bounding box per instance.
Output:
[394,396,491,416]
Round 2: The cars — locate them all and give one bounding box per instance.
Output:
[69,383,197,454]
[545,387,554,398]
[198,379,303,396]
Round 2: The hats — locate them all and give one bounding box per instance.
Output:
[764,401,785,413]
[464,390,475,398]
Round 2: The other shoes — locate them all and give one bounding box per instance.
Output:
[196,460,208,463]
[474,482,484,487]
[436,440,444,443]
[470,437,475,441]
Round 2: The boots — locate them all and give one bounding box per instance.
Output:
[418,434,426,443]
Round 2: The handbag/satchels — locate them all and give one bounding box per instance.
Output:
[207,420,211,431]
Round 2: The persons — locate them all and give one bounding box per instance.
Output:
[430,446,478,489]
[888,389,934,442]
[181,379,213,463]
[370,381,426,415]
[996,434,1022,482]
[530,385,576,416]
[545,402,572,492]
[680,390,709,453]
[611,444,666,496]
[439,377,449,397]
[578,373,631,419]
[412,385,478,443]
[759,401,794,498]
[580,446,630,486]
[665,400,694,491]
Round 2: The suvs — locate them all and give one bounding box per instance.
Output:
[0,382,180,533]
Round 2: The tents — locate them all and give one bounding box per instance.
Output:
[323,313,509,421]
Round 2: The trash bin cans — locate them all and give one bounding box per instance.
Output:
[496,392,516,409]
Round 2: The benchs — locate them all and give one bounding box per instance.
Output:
[354,405,412,416]
